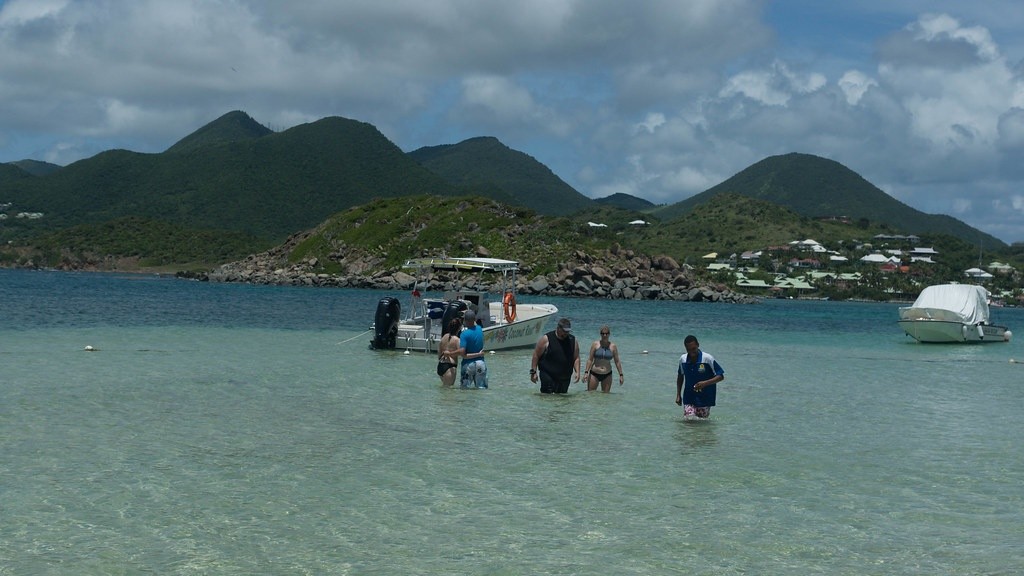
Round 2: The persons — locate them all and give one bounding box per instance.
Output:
[442,310,489,389]
[582,324,624,393]
[676,335,724,418]
[530,318,580,394]
[437,319,484,386]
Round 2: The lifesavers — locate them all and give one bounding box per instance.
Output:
[504,293,517,323]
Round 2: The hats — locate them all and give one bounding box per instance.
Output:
[465,311,475,321]
[559,322,572,331]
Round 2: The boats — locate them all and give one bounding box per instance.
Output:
[367,252,558,353]
[896,281,1012,345]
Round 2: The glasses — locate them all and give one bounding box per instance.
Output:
[600,333,610,335]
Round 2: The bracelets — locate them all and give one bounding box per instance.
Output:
[620,374,623,376]
[585,371,588,373]
[530,369,536,375]
